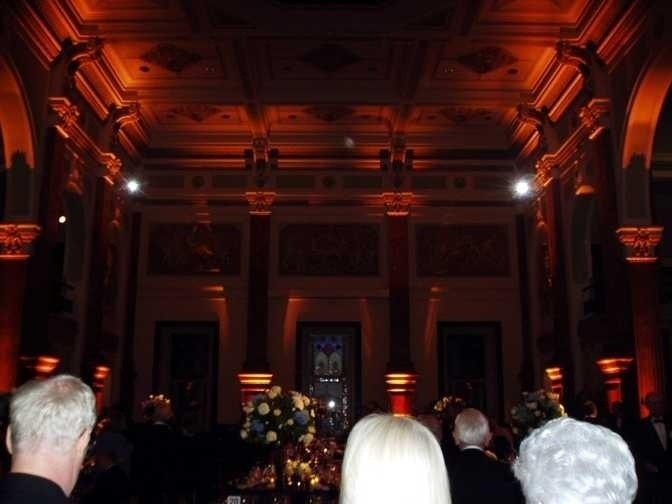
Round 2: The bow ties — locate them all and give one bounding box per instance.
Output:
[655,420,664,423]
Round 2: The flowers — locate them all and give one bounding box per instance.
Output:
[239,386,320,448]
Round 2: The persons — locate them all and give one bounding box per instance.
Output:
[0,373,194,503]
[339,388,672,504]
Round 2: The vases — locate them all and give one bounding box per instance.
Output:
[271,445,290,496]
